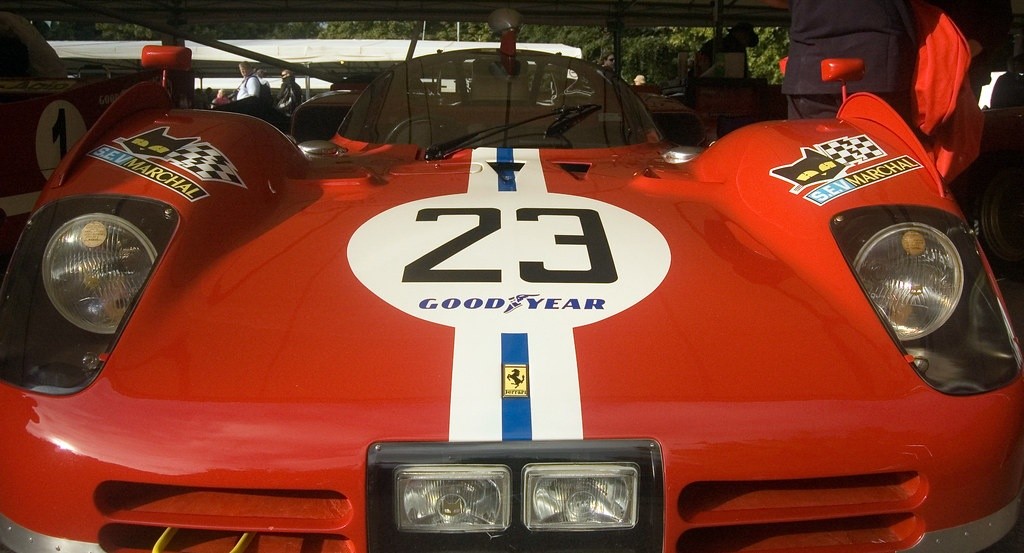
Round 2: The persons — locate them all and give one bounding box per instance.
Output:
[196,62,302,114]
[600,0,1024,195]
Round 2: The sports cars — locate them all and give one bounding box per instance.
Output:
[0,0,1024,553]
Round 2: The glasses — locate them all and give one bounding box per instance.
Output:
[609,58,615,61]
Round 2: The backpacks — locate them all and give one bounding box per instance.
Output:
[245,76,271,102]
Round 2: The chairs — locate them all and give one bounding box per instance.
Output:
[382,112,463,151]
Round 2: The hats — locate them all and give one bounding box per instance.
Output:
[634,75,646,83]
